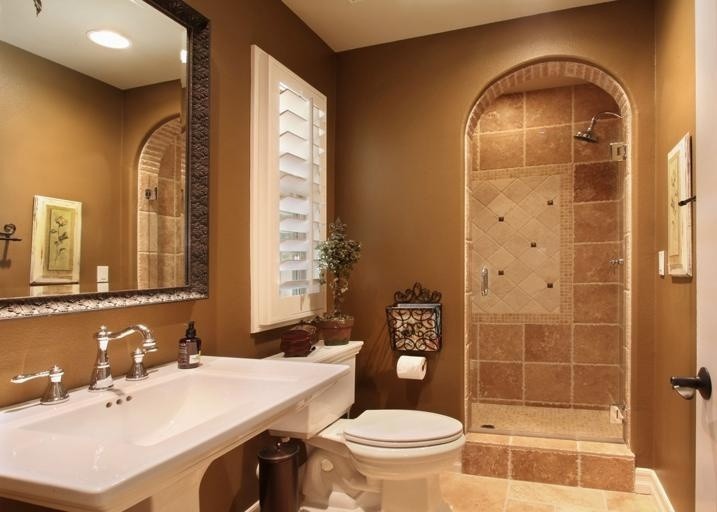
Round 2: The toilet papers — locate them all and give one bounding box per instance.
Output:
[397,355,427,380]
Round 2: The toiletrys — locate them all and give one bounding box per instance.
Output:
[178,322,202,368]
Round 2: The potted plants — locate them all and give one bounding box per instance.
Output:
[311,216,363,345]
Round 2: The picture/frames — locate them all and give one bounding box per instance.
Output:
[28,194,84,287]
[664,131,694,280]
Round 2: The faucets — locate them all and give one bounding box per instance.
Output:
[88,324,158,391]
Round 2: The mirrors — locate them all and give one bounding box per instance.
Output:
[0,0,212,320]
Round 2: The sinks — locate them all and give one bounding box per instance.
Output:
[0,355,350,511]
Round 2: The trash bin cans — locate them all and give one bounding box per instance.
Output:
[257,441,299,512]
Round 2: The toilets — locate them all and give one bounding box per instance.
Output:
[263,341,466,512]
